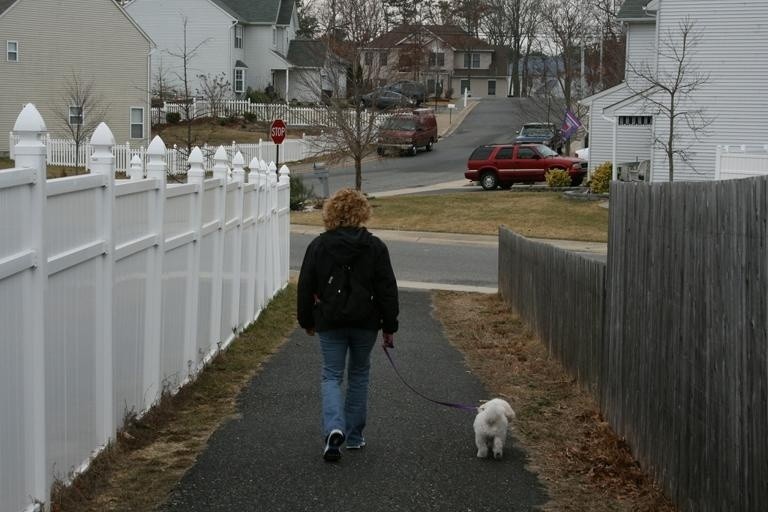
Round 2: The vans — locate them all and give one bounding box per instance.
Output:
[377,109,438,158]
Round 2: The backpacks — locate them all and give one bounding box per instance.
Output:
[314,232,380,330]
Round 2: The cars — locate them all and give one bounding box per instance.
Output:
[573,148,588,161]
[348,80,427,108]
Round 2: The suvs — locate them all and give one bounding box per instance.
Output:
[464,142,588,192]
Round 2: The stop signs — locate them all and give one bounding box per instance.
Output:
[270,119,287,145]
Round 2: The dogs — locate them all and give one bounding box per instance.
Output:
[472,398,516,459]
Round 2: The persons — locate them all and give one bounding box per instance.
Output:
[294,188,402,461]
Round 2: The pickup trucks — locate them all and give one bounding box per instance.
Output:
[513,122,565,153]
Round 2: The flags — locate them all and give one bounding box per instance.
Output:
[558,108,583,144]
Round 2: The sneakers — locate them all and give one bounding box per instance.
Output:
[322,428,345,462]
[346,437,365,449]
